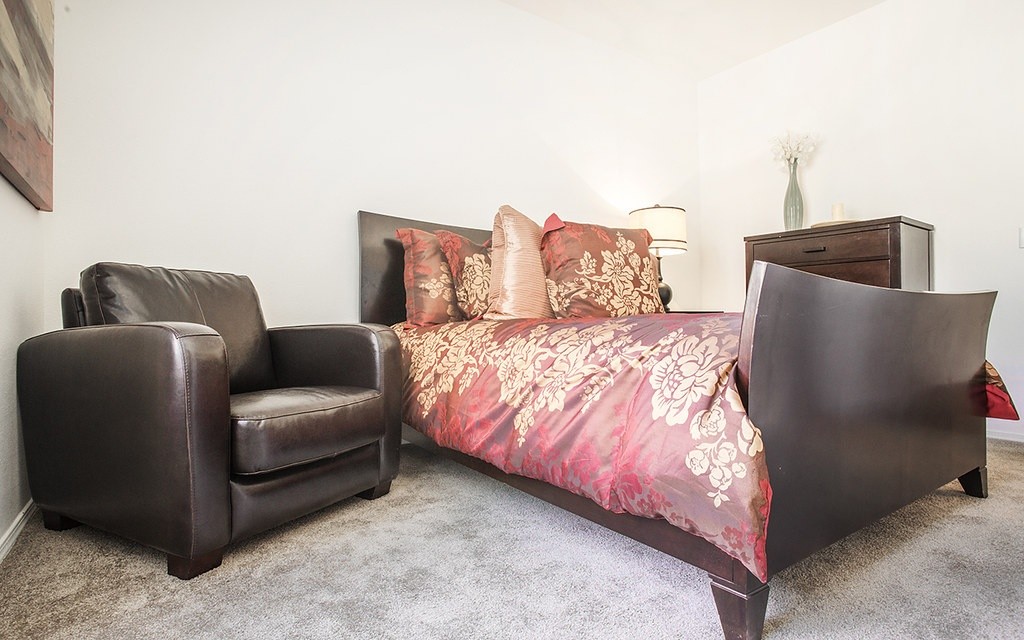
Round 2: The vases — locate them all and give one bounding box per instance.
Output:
[783,158,804,229]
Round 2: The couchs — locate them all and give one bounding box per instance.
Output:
[21,263,398,579]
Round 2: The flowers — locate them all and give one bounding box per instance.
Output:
[772,131,818,166]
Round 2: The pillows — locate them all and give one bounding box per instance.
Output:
[483,207,555,320]
[541,213,665,318]
[393,228,494,326]
[437,228,502,321]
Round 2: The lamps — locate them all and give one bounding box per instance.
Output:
[627,203,688,311]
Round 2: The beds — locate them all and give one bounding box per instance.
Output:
[358,205,1019,639]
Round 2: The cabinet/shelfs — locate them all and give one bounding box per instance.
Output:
[742,215,935,301]
